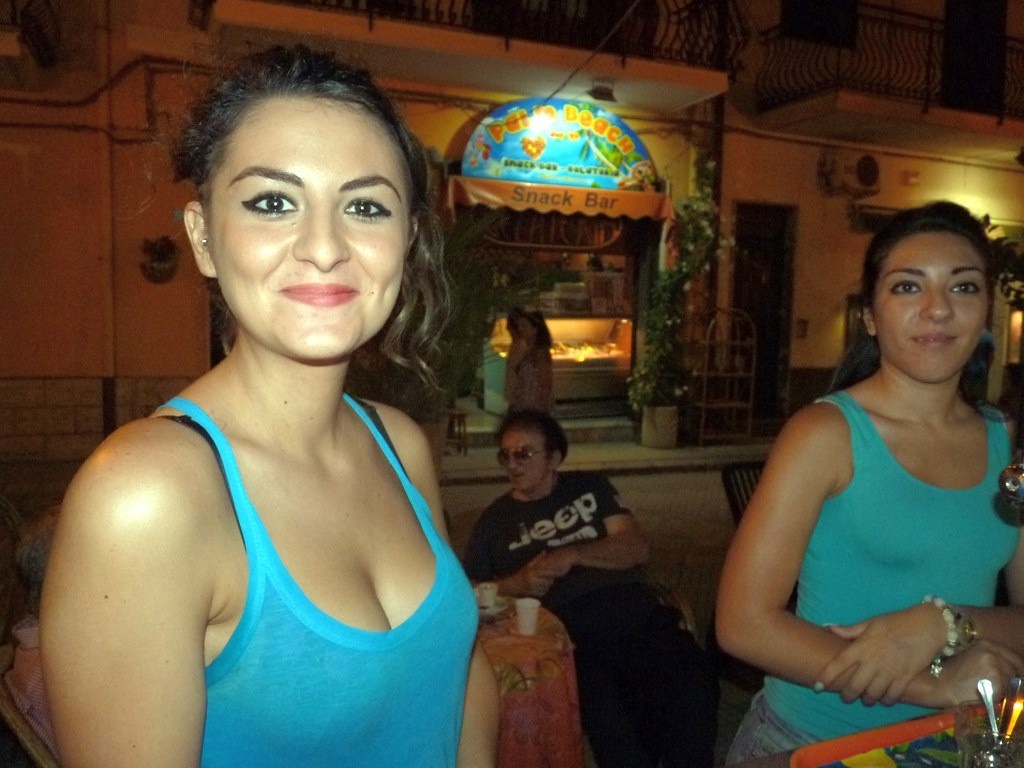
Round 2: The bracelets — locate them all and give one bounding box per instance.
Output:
[920,594,959,678]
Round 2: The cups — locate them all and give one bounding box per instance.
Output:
[479,582,497,606]
[515,598,541,636]
[957,696,1024,768]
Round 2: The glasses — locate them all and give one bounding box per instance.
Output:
[497,445,552,467]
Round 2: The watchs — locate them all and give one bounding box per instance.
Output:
[950,601,977,651]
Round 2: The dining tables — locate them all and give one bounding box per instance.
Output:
[11,591,585,768]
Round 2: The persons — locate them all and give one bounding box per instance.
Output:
[37,47,502,768]
[4,500,60,762]
[507,312,555,409]
[715,202,1024,766]
[479,316,512,410]
[963,326,994,400]
[460,404,720,768]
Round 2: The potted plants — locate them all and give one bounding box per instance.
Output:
[365,203,585,481]
[626,161,719,451]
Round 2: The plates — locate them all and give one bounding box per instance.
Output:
[476,595,508,613]
